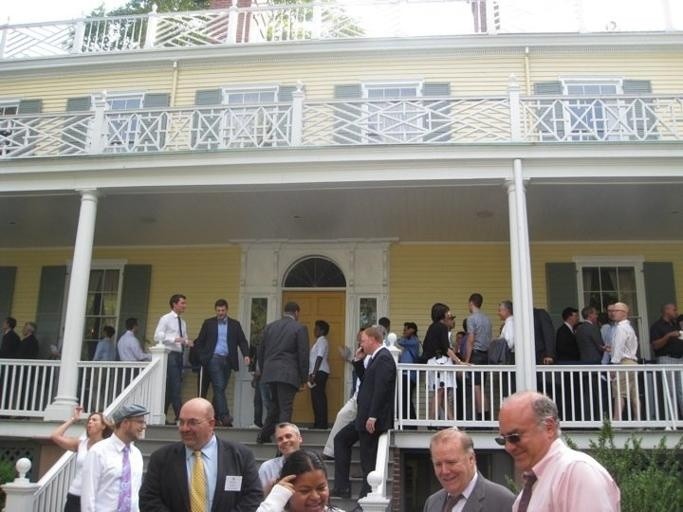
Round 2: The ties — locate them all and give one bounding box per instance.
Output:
[444,495,463,512]
[517,476,536,512]
[190,451,205,512]
[117,447,130,512]
[177,317,183,351]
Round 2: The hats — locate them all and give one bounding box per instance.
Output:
[112,404,150,424]
[447,313,456,320]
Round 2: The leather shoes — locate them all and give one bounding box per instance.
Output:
[222,416,233,426]
[328,488,350,499]
[256,432,271,442]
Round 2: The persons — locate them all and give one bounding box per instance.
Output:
[321,323,387,461]
[553,301,682,429]
[258,422,304,492]
[49,402,112,512]
[137,397,266,512]
[419,428,518,512]
[0,486,8,511]
[254,450,346,512]
[80,404,152,512]
[153,295,331,430]
[2,317,153,422]
[338,291,557,429]
[493,391,621,512]
[327,328,395,500]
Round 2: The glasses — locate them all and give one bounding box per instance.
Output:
[128,420,146,425]
[176,418,209,427]
[494,434,520,445]
